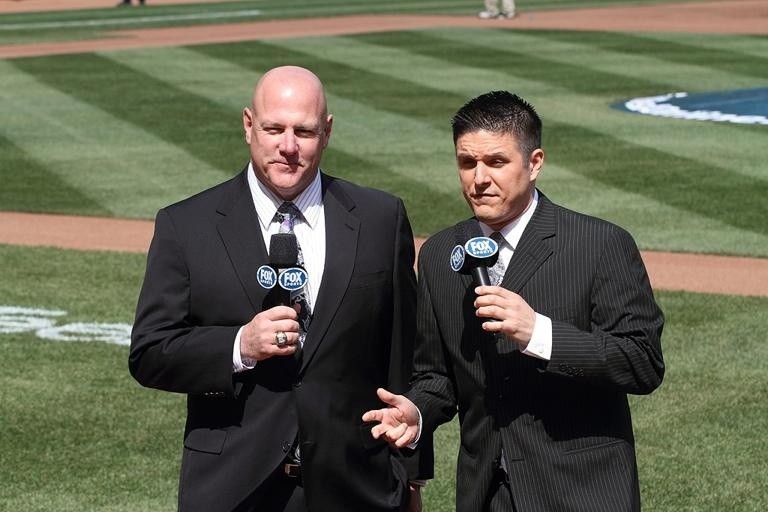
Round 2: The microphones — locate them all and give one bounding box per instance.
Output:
[257,233,308,307]
[450,218,502,338]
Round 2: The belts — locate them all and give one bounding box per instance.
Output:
[276,462,302,477]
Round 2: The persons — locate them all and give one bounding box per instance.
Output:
[117,0,146,7]
[478,0,517,19]
[362,93,665,512]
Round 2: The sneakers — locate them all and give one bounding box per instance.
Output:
[479,11,515,19]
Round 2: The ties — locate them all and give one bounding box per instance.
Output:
[275,204,312,359]
[486,232,505,286]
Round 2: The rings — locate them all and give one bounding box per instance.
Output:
[128,66,433,512]
[275,331,287,348]
